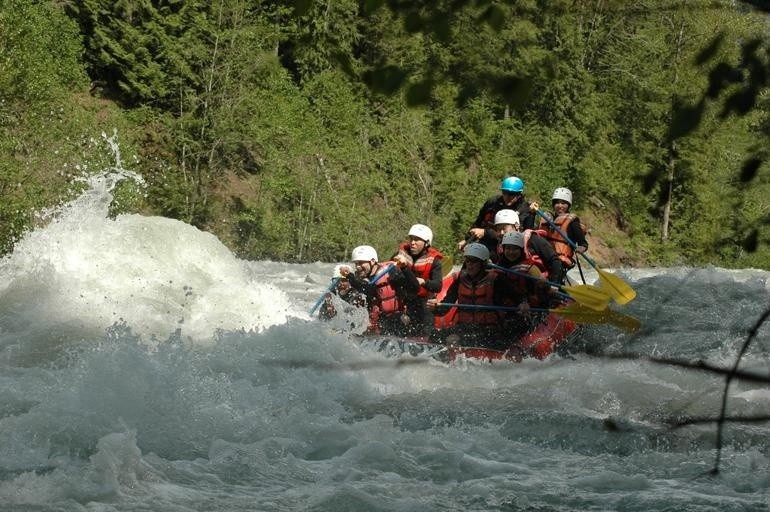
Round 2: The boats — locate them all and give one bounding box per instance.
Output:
[320,279,585,362]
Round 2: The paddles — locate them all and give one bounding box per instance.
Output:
[440,233,478,278]
[436,301,610,325]
[487,264,610,311]
[534,206,636,305]
[555,291,641,333]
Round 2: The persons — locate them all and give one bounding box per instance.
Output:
[390,221,443,304]
[453,176,536,264]
[318,263,365,322]
[351,244,421,335]
[533,187,588,275]
[489,208,563,294]
[426,242,530,349]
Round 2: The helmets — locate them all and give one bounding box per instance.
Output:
[350,245,378,263]
[501,231,524,249]
[463,243,489,263]
[500,176,523,192]
[552,187,572,206]
[409,224,433,247]
[332,265,354,279]
[494,209,520,227]
